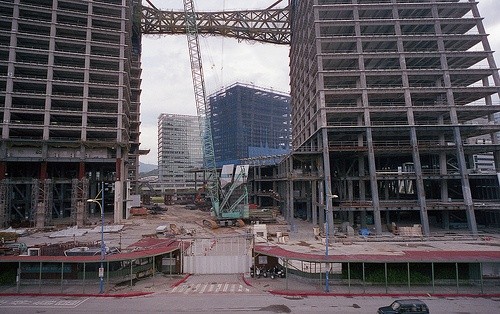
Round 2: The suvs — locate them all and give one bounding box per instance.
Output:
[376,299,429,314]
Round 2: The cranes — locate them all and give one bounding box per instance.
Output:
[184,1,251,228]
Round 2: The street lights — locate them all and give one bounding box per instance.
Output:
[87,199,104,293]
[324,195,338,293]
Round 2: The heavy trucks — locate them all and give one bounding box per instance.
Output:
[195,188,213,212]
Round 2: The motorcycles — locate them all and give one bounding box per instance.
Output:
[248,264,284,279]
